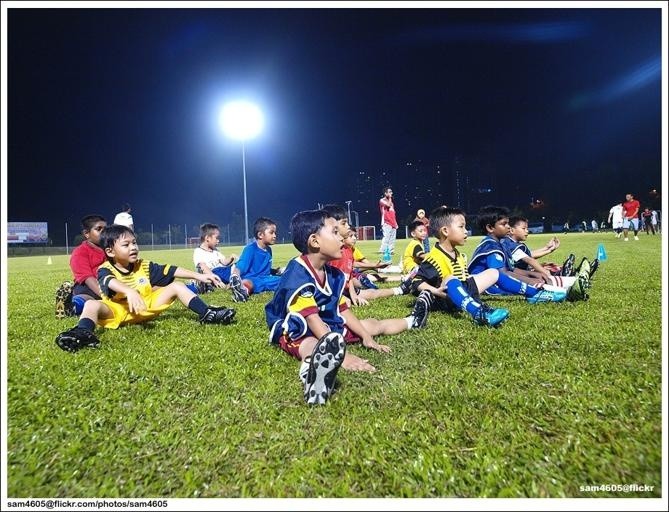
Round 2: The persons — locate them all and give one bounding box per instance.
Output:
[529,237,576,278]
[377,188,398,255]
[411,209,431,253]
[114,204,136,234]
[502,215,600,285]
[411,207,567,327]
[311,205,414,306]
[402,223,428,277]
[263,207,434,407]
[53,225,237,350]
[186,216,237,294]
[345,246,417,280]
[466,202,591,301]
[621,193,640,241]
[55,214,215,320]
[342,225,380,292]
[226,217,284,304]
[608,202,624,238]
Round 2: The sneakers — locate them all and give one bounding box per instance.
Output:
[634,236,640,241]
[200,305,236,326]
[477,304,509,326]
[361,276,379,289]
[561,255,574,277]
[401,266,420,296]
[624,237,629,242]
[194,275,212,294]
[590,259,599,277]
[568,277,589,300]
[574,257,590,280]
[407,288,433,330]
[229,273,248,302]
[300,332,346,407]
[56,281,75,320]
[528,290,566,304]
[57,326,100,352]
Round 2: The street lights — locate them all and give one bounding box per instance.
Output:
[218,100,265,244]
